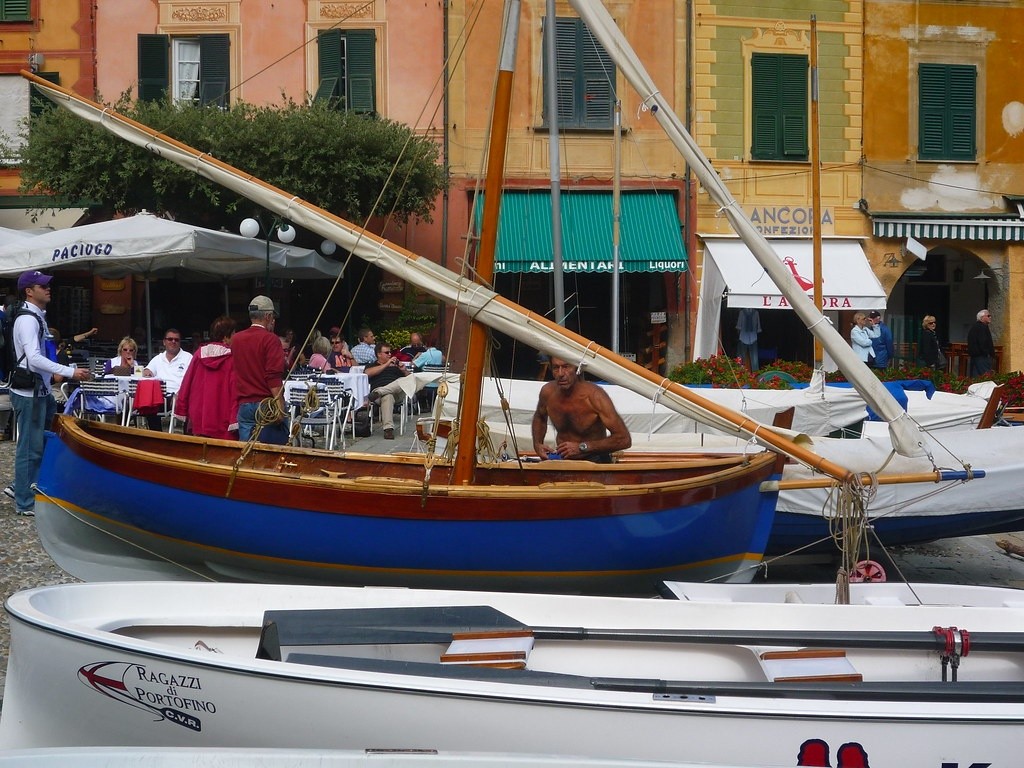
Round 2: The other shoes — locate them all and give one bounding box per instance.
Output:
[16,507,35,516]
[384,428,393,439]
[368,392,379,402]
[5,481,16,499]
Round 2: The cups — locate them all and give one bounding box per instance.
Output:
[134,365,144,377]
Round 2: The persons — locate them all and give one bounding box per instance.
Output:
[143,329,193,432]
[350,327,378,364]
[104,337,141,374]
[39,328,98,362]
[400,331,442,367]
[176,314,238,441]
[967,309,996,378]
[273,327,306,373]
[920,315,947,371]
[3,270,89,516]
[849,311,894,370]
[302,326,358,368]
[363,341,430,438]
[233,295,289,444]
[531,355,631,463]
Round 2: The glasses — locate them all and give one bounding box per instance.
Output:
[122,349,135,352]
[165,338,179,342]
[380,351,391,354]
[988,315,991,318]
[331,342,341,345]
[928,322,936,325]
[35,283,49,289]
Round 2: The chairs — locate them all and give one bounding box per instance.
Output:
[60,351,450,451]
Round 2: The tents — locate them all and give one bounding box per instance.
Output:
[0,210,346,278]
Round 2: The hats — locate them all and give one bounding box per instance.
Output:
[329,327,340,333]
[248,295,280,319]
[868,311,880,318]
[17,270,53,290]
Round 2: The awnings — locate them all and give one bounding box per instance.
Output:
[470,189,690,274]
[699,238,887,308]
[865,209,1024,242]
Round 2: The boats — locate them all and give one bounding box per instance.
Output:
[414,370,1024,542]
[0,568,1024,768]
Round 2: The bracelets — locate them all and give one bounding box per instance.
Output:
[350,357,354,359]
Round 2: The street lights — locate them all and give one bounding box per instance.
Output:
[237,214,296,301]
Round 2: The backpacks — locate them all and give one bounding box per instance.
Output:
[351,407,371,436]
[0,309,43,382]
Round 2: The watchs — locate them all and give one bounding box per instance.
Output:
[578,442,589,454]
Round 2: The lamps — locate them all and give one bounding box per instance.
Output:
[900,237,928,261]
[973,267,1003,279]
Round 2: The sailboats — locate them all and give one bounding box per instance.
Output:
[41,1,986,584]
